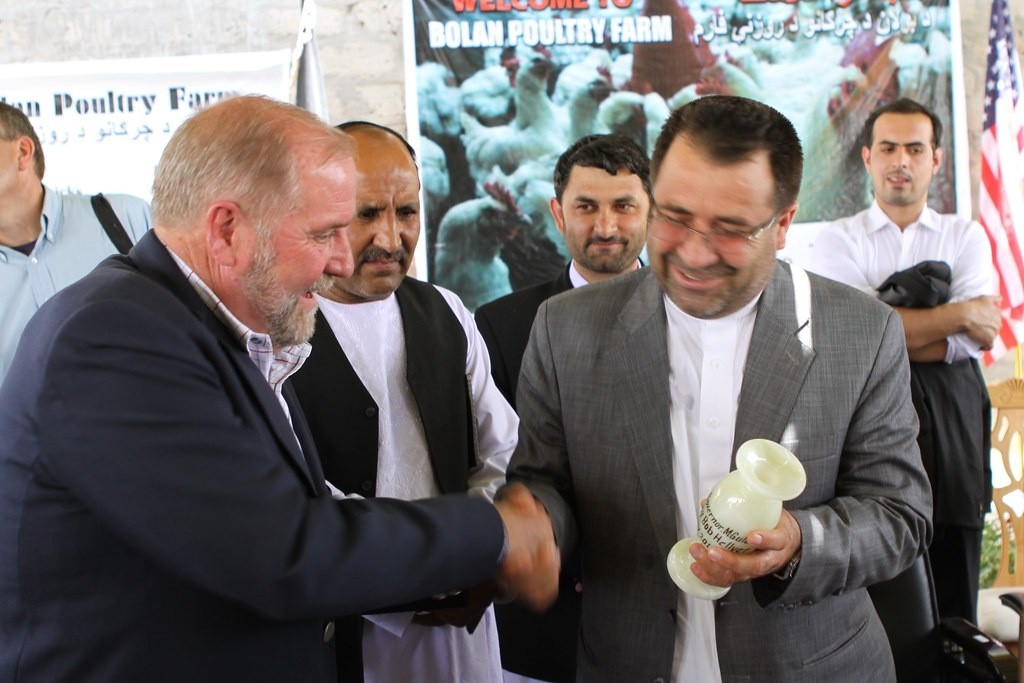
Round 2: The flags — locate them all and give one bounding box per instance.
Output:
[967,0,1024,369]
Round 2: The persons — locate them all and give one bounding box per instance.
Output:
[0,99,559,683]
[0,103,157,390]
[811,99,1003,632]
[294,94,932,683]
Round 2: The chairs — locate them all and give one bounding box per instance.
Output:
[986,379,1024,588]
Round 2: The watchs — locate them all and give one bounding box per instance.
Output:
[772,549,802,580]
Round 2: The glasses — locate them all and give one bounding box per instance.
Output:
[649,188,777,253]
